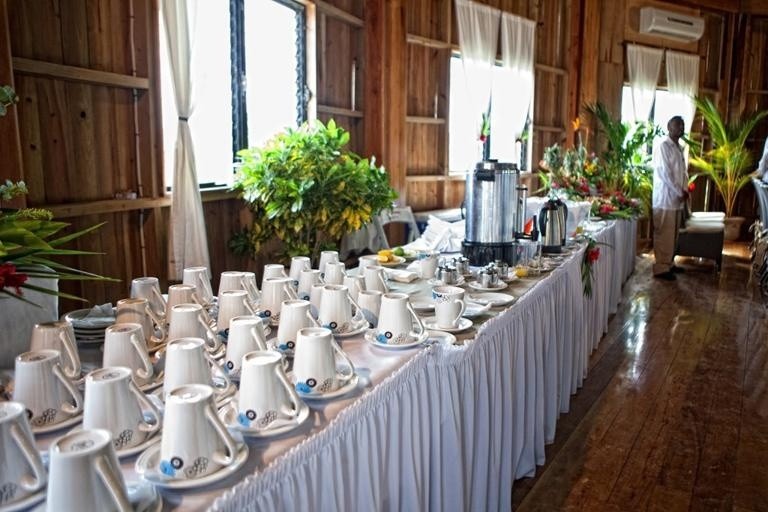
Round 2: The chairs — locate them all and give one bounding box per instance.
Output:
[671,192,726,274]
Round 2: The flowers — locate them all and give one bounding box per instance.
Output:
[539,99,665,220]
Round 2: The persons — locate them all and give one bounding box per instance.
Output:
[652,116,688,282]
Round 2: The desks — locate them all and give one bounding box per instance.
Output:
[0,214,638,512]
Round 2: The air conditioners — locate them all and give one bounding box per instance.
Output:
[639,7,706,43]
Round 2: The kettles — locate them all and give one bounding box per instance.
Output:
[539,201,569,253]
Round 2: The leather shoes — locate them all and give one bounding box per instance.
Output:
[670,266,687,275]
[654,271,677,282]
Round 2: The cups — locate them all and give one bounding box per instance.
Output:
[161,337,230,402]
[512,242,528,276]
[224,315,268,379]
[82,366,162,450]
[373,293,425,345]
[46,429,134,512]
[431,286,468,328]
[29,321,83,379]
[115,297,165,349]
[272,299,320,356]
[166,303,220,352]
[13,349,83,429]
[315,285,365,332]
[155,383,237,481]
[525,241,541,276]
[102,322,154,386]
[233,350,301,430]
[1,399,49,504]
[1,251,517,316]
[292,328,354,397]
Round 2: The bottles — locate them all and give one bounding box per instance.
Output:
[531,215,539,242]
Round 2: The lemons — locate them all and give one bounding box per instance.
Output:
[378,246,404,262]
[515,267,530,277]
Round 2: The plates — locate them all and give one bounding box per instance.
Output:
[363,328,429,350]
[218,399,310,437]
[28,475,165,512]
[25,412,83,434]
[412,316,473,332]
[266,337,294,361]
[285,364,359,400]
[65,394,161,458]
[133,424,250,491]
[78,378,157,393]
[150,378,238,409]
[310,321,370,339]
[420,330,458,348]
[2,448,49,512]
[540,244,577,272]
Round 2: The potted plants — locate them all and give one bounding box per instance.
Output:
[686,93,768,240]
